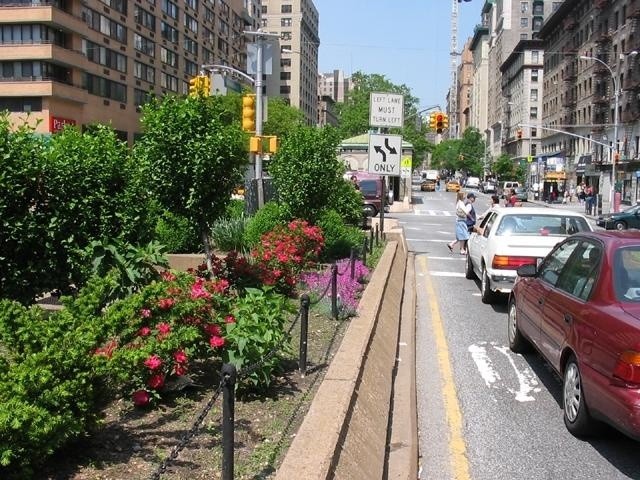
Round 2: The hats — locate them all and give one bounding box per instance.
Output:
[466,192,477,199]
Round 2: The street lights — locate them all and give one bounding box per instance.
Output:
[580,51,638,214]
[430,111,449,134]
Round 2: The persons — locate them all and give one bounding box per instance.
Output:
[532,182,540,201]
[562,181,594,214]
[435,167,518,255]
[351,176,360,191]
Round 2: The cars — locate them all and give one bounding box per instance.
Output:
[412,169,480,193]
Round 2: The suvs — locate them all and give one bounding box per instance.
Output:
[342,171,394,217]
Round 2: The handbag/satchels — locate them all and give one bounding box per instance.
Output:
[456,208,466,219]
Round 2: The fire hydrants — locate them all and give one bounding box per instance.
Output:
[614,193,620,212]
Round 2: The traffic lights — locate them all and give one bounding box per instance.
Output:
[189,75,210,97]
[458,155,464,160]
[517,130,522,138]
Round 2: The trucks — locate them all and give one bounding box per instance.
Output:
[479,181,528,202]
